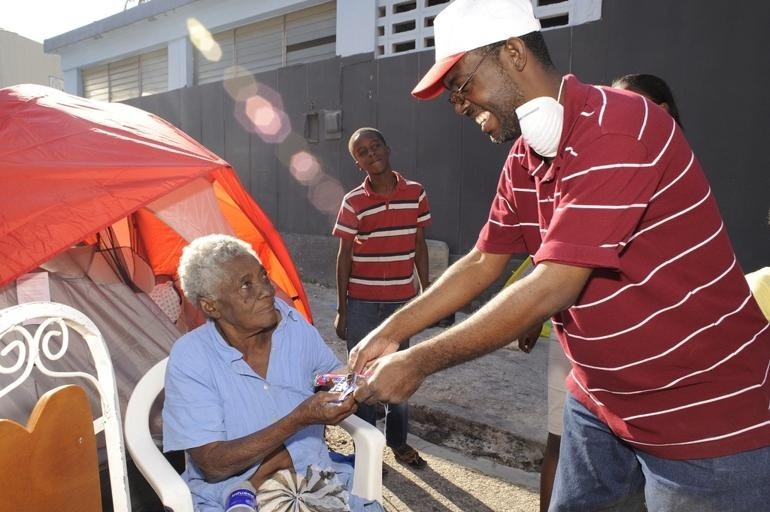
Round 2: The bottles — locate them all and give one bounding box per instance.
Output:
[224,481,259,512]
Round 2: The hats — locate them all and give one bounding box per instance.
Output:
[410,0,542,103]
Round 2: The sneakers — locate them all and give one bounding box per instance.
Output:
[391,443,429,468]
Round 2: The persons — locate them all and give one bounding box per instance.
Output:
[517,70,683,510]
[331,127,433,474]
[342,0,770,511]
[158,233,372,511]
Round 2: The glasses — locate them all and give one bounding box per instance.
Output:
[447,43,508,104]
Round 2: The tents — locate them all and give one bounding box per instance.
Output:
[0,80,317,511]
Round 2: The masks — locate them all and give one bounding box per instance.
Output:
[512,96,564,157]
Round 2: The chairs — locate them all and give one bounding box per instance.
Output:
[125,357,386,512]
[2,300,132,512]
